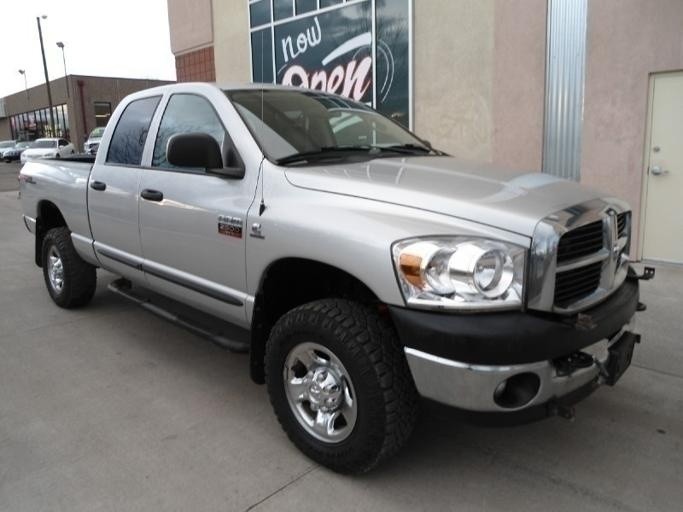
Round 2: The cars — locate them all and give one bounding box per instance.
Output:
[83,126,107,156]
[0,137,75,165]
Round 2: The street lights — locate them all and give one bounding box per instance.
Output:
[36,15,56,137]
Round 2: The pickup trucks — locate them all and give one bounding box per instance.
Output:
[17,82,655,477]
